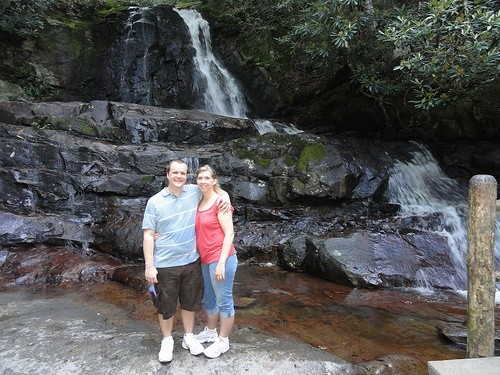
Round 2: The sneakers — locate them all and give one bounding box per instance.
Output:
[196,326,230,358]
[158,332,204,364]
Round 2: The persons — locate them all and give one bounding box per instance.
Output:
[142,160,231,363]
[154,165,238,359]
